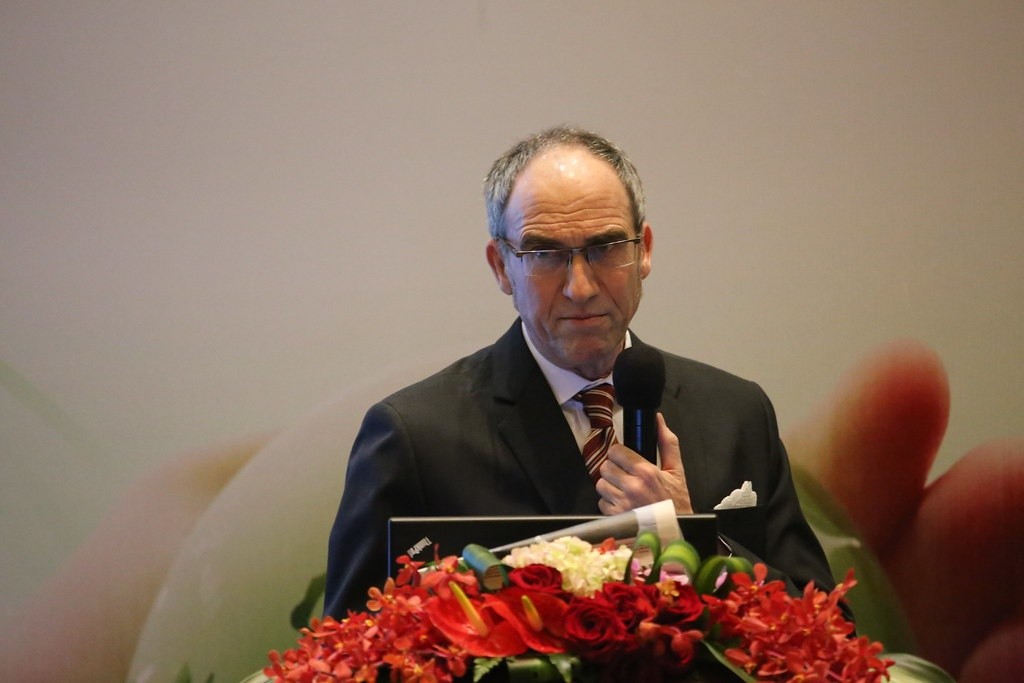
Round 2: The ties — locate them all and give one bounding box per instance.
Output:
[573,383,619,486]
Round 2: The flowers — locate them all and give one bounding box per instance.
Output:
[262,536,895,683]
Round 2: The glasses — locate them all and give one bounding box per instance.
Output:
[495,221,646,278]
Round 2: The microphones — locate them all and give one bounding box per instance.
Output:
[613,345,666,467]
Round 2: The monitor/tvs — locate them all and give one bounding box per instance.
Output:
[387,512,720,587]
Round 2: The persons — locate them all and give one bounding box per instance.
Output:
[322,126,860,683]
